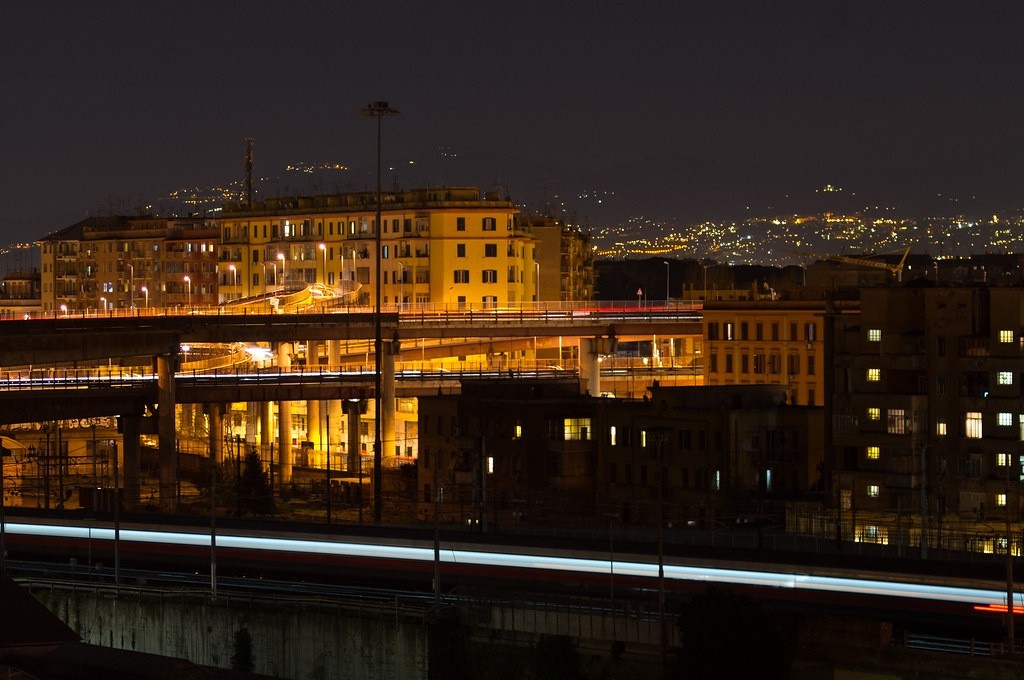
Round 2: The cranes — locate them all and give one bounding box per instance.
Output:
[773,246,913,282]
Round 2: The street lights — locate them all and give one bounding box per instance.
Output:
[356,97,400,521]
